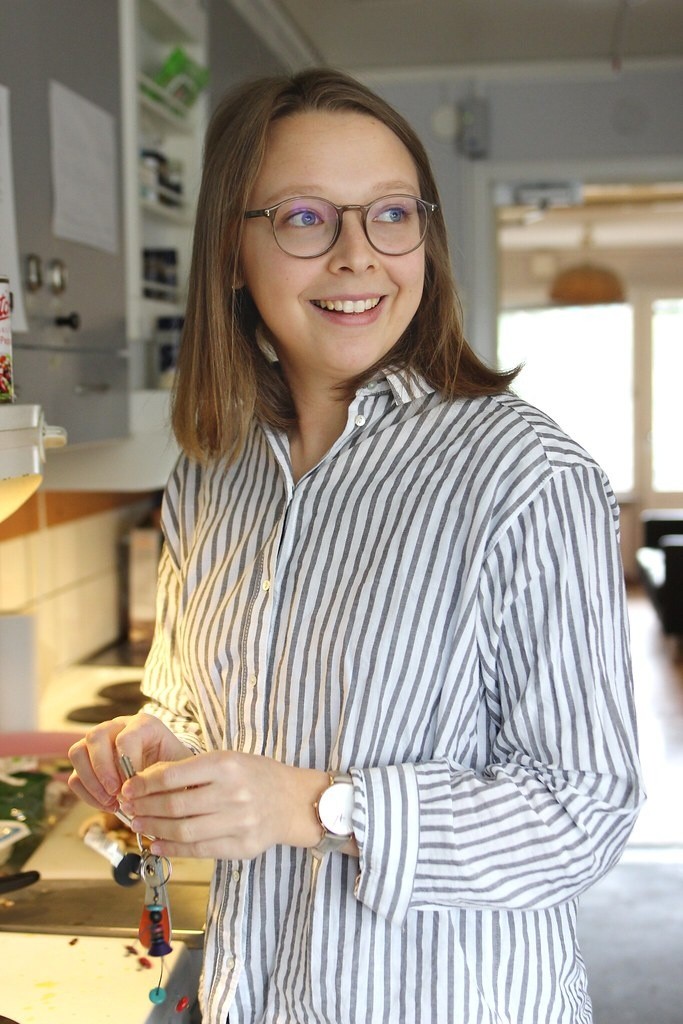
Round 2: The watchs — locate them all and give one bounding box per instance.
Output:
[310,771,355,859]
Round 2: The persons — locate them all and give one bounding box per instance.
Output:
[67,67,642,1023]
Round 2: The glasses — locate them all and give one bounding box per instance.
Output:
[244,194,438,259]
